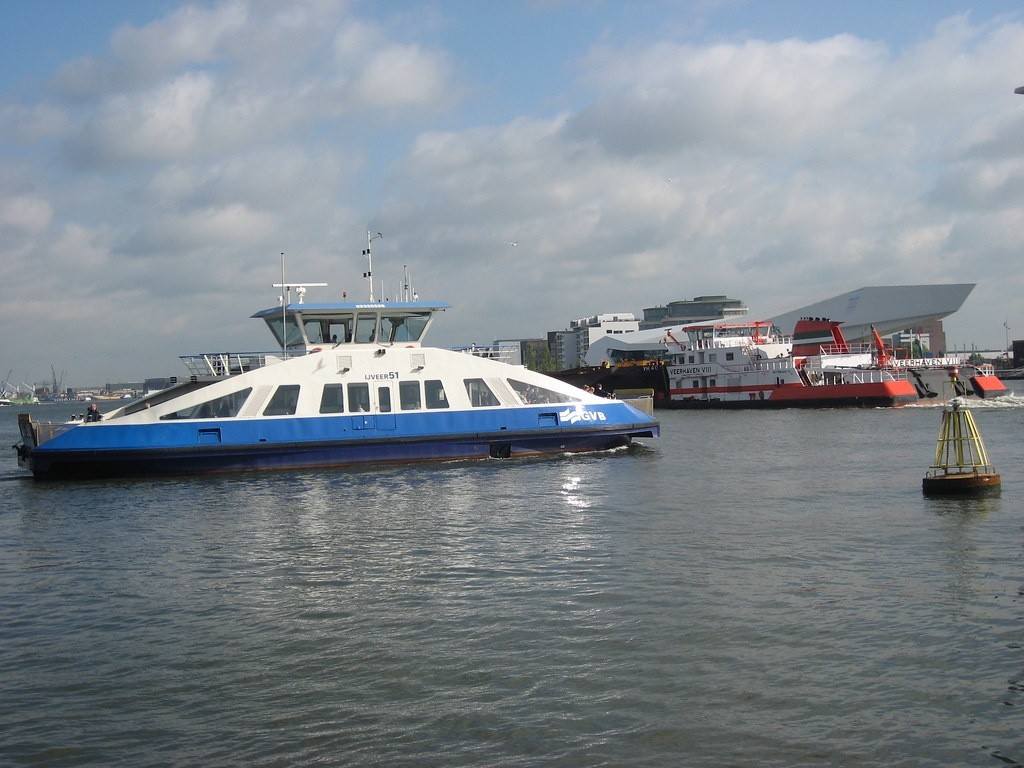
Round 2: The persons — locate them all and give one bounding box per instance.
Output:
[582,383,609,398]
[87,404,103,422]
[469,343,493,357]
[346,330,352,342]
[331,335,337,343]
[369,329,375,342]
[214,399,229,418]
[413,401,420,409]
[357,404,365,412]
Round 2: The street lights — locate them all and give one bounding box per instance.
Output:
[1003,320,1012,353]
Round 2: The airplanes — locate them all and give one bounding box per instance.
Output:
[507,241,519,247]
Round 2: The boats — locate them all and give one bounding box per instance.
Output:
[0,386,147,407]
[14,223,661,485]
[658,316,1010,414]
